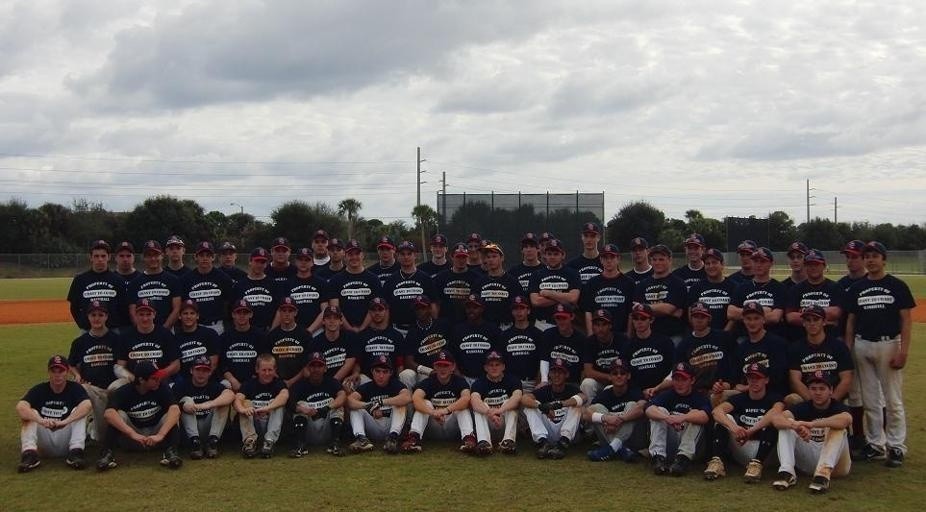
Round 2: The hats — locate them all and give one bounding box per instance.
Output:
[88,291,825,320]
[191,354,214,372]
[609,358,631,370]
[807,370,833,389]
[432,349,454,366]
[744,362,771,379]
[371,355,393,371]
[46,354,69,373]
[548,357,568,373]
[87,218,887,263]
[670,362,697,379]
[132,359,166,380]
[306,351,327,367]
[482,350,504,365]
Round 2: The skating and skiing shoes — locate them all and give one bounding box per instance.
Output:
[670,451,695,476]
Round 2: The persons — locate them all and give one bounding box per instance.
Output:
[15,222,918,493]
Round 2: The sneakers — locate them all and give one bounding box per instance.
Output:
[769,471,800,491]
[188,433,423,460]
[158,445,185,470]
[807,474,832,494]
[586,445,617,463]
[477,440,493,455]
[858,443,886,462]
[548,436,576,459]
[16,450,43,474]
[459,435,477,453]
[618,446,643,464]
[96,452,120,472]
[64,447,89,472]
[535,438,553,458]
[885,444,905,468]
[702,455,729,480]
[741,458,764,486]
[500,439,517,454]
[650,454,670,477]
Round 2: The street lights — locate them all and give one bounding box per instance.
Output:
[229,201,244,215]
